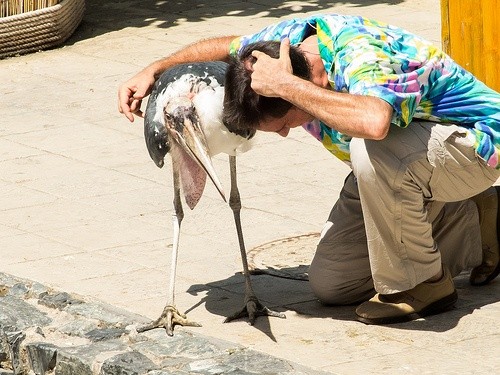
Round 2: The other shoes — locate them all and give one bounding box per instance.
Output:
[354,263,458,324]
[469,185,500,287]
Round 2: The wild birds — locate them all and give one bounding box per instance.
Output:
[136,61,286,337]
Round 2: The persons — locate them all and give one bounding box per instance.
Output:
[118,13,499,324]
